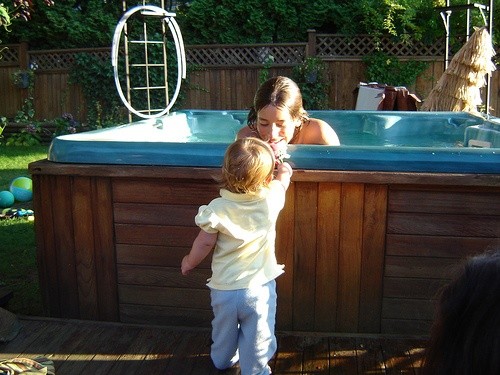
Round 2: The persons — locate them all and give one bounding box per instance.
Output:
[239,76,341,146]
[182,139,292,375]
[421,249,500,375]
